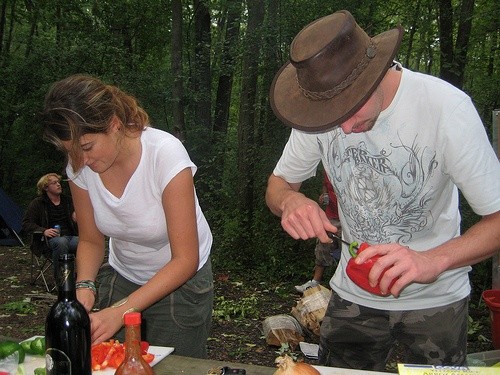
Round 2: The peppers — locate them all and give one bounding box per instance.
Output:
[346,241,401,295]
[91,339,155,370]
[0,336,47,375]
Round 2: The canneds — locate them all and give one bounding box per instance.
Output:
[54,225,60,236]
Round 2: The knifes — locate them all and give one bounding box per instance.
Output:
[327,231,359,249]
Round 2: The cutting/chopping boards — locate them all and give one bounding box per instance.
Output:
[310,364,398,375]
[0,335,176,375]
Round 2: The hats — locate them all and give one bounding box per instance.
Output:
[268,9,403,133]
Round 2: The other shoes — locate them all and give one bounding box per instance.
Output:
[293,279,319,294]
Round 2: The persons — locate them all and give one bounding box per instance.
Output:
[294,168,342,296]
[30,74,213,359]
[265,9,500,371]
[24,173,79,292]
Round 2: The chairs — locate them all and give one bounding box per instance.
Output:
[0,188,58,294]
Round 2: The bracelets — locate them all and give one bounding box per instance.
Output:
[74,279,97,296]
[111,296,142,327]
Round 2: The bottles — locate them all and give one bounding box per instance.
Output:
[114,312,155,375]
[44,253,92,375]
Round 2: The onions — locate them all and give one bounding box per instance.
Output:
[268,354,319,375]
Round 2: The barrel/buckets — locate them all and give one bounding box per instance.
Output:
[481,289,500,350]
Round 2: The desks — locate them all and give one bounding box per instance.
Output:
[148,355,381,375]
